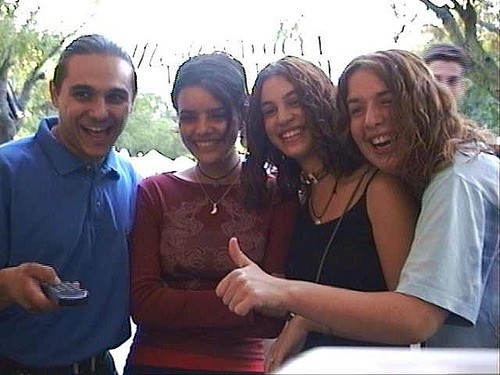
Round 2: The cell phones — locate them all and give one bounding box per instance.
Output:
[42,281,89,307]
